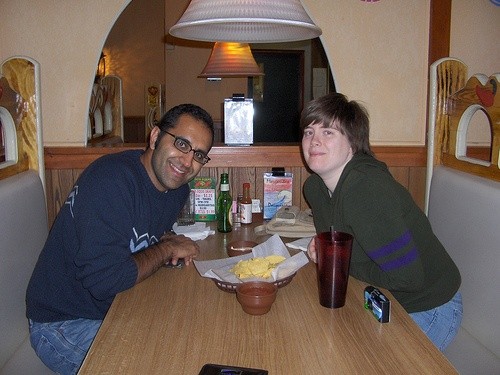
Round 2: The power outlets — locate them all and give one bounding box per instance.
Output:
[198,41,266,78]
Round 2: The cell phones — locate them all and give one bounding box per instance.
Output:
[164,258,185,270]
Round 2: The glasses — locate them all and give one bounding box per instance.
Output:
[160,129,211,165]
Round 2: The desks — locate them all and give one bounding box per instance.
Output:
[77,212,461,375]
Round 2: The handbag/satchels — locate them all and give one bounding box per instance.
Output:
[253,206,317,238]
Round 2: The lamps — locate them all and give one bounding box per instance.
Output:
[97,52,107,76]
[168,0,322,42]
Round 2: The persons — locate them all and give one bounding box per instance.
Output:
[25,104,214,375]
[299,92,462,352]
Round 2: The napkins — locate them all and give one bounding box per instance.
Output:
[171,221,216,241]
[285,238,313,251]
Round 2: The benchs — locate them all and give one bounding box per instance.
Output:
[427,167,500,375]
[1,170,57,375]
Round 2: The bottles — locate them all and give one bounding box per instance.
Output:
[215,174,233,233]
[240,183,252,224]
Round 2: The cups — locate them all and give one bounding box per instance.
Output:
[235,281,278,316]
[314,231,354,309]
[226,240,258,258]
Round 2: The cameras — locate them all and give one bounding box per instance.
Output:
[363,285,392,324]
